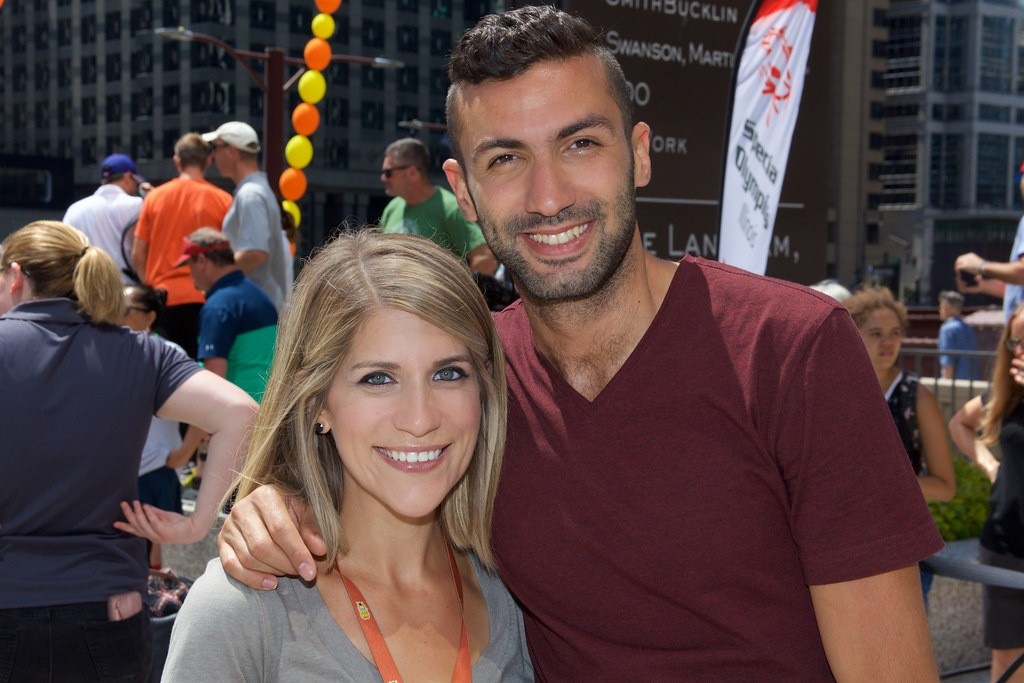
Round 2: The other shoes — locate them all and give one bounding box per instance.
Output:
[176,461,197,486]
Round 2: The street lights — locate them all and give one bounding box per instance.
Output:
[155,25,407,207]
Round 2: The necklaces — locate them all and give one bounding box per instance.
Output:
[334,506,474,683]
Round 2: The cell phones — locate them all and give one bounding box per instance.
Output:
[960,268,977,287]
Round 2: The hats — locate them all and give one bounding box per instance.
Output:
[202,122,262,154]
[173,236,230,269]
[100,153,146,186]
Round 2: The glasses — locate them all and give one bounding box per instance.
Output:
[1006,336,1024,353]
[380,165,423,178]
[209,141,228,150]
[123,302,154,316]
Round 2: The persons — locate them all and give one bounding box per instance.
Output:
[949,301,1023,682]
[202,121,294,316]
[118,284,189,624]
[172,226,279,513]
[954,162,1023,327]
[62,154,153,290]
[216,5,945,683]
[843,284,956,619]
[132,133,234,475]
[160,227,534,683]
[0,219,260,683]
[375,138,501,275]
[937,290,979,413]
